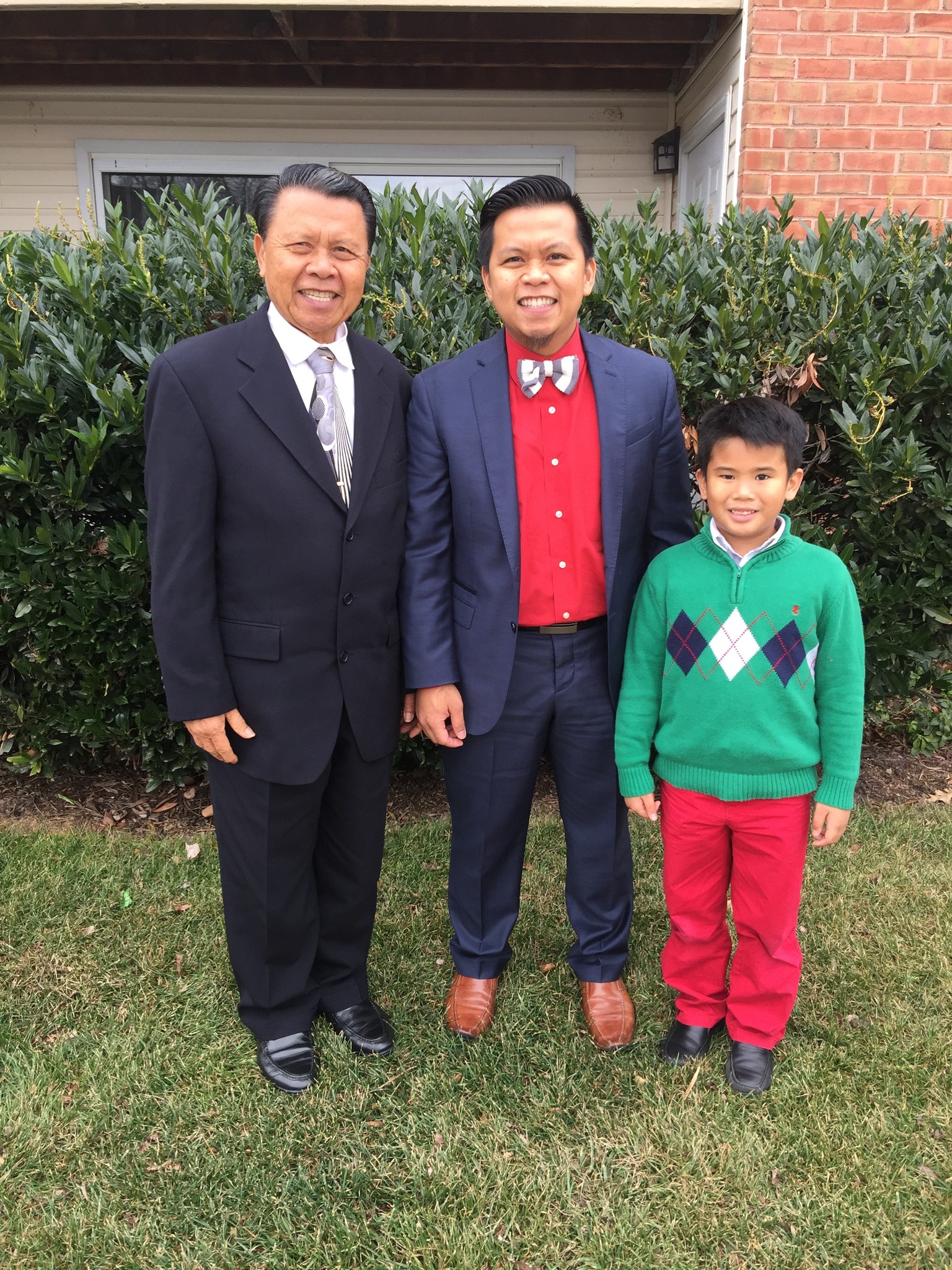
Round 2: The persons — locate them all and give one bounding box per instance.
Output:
[617,397,864,1091]
[141,165,419,1093]
[398,173,701,1051]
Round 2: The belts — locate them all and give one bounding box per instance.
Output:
[523,613,608,636]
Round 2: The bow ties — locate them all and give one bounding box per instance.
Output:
[517,356,580,399]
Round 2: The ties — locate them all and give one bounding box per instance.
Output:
[307,349,354,507]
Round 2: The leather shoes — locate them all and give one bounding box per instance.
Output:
[726,1034,773,1095]
[256,1030,316,1093]
[328,999,397,1059]
[580,974,636,1047]
[443,971,499,1041]
[661,1015,710,1063]
